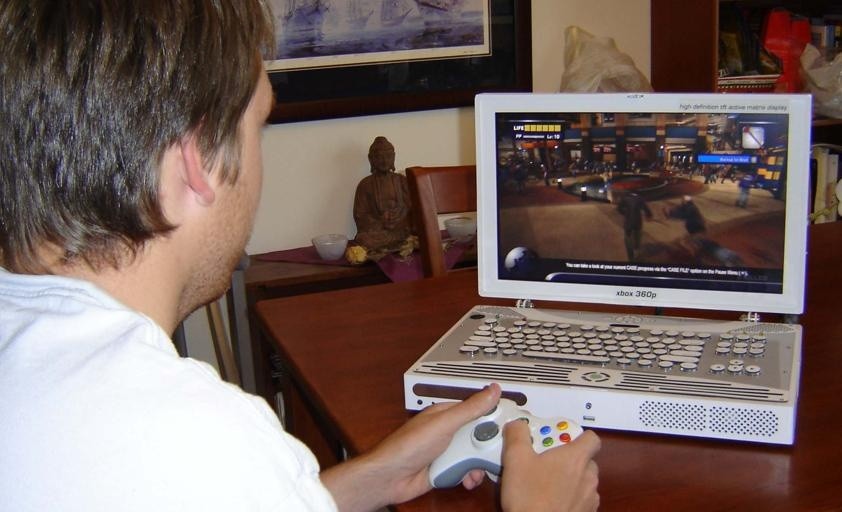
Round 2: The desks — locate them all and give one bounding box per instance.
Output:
[258,226,839,507]
[242,230,477,381]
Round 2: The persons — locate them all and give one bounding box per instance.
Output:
[0,0,602,511]
[353,137,419,261]
[497,113,753,258]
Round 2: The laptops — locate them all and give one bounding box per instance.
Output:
[404,92,813,447]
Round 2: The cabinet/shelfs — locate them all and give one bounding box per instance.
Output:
[641,3,842,225]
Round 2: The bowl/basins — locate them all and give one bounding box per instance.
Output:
[444,217,479,242]
[313,234,346,261]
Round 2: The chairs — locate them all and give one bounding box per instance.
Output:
[405,156,474,274]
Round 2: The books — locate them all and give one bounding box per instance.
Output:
[717,15,842,89]
[811,146,839,225]
